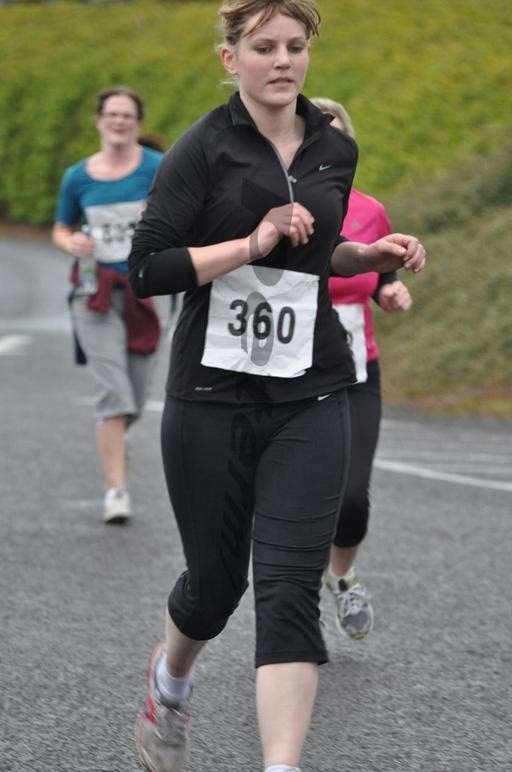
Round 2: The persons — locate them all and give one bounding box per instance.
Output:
[48,84,183,526]
[305,96,412,642]
[127,1,427,772]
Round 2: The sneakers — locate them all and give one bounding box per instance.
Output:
[323,563,373,641]
[135,642,191,772]
[102,488,130,523]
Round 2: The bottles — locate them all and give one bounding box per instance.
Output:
[77,224,99,296]
